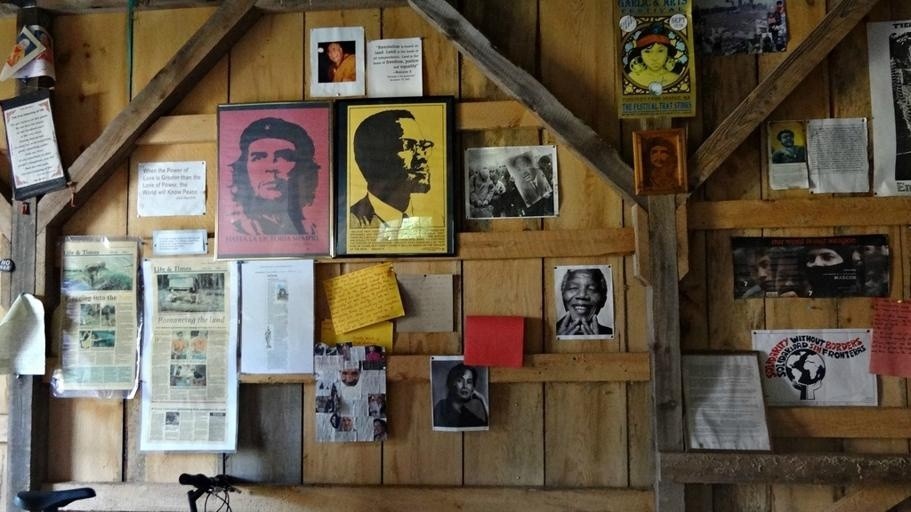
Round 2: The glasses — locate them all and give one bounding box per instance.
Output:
[397,137,435,152]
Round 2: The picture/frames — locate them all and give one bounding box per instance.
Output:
[335,96,457,257]
[214,99,336,261]
[632,128,688,196]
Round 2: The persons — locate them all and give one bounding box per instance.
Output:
[315,344,386,442]
[433,362,487,427]
[224,117,320,237]
[628,28,679,89]
[696,1,786,53]
[744,242,887,295]
[350,110,433,227]
[771,128,805,165]
[557,269,611,336]
[323,40,354,82]
[643,136,678,190]
[466,155,552,218]
